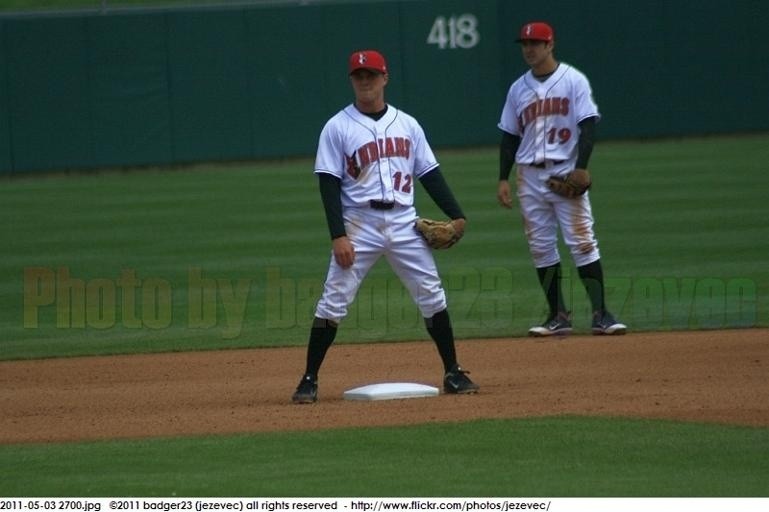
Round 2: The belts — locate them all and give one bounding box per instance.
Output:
[529,160,563,168]
[370,200,395,210]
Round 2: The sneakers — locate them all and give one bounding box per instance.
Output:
[528,312,573,336]
[293,375,317,402]
[592,310,627,334]
[444,364,479,393]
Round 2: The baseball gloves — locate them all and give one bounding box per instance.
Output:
[413,217,461,250]
[546,169,591,197]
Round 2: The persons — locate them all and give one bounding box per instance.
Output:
[283,48,482,405]
[495,23,628,338]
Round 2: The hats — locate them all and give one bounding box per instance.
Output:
[349,51,386,74]
[515,23,553,41]
